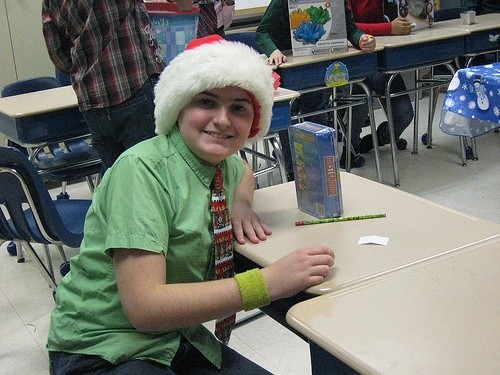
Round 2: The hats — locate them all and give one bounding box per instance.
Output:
[154,34,273,145]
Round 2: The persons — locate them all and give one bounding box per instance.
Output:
[41,0,425,186]
[45,35,335,375]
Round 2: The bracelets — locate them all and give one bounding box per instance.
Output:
[234,268,270,312]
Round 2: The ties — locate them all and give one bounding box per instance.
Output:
[210,168,235,348]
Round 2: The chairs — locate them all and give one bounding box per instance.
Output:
[222,31,265,55]
[1,77,102,199]
[0,145,92,294]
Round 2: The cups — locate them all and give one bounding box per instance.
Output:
[460,10,476,25]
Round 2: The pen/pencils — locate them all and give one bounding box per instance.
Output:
[295,213,386,226]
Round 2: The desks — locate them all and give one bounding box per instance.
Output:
[0,12,500,375]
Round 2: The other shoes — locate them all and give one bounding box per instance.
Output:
[360,130,384,154]
[340,137,361,169]
